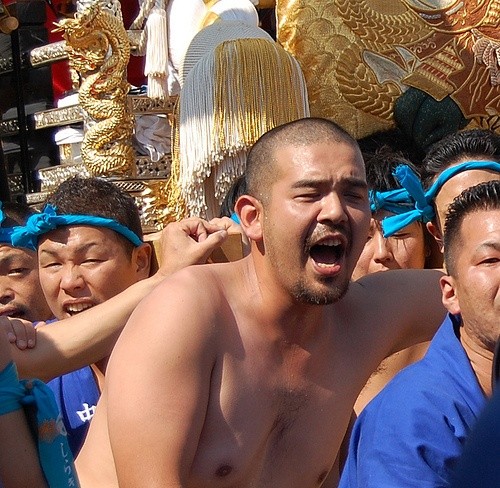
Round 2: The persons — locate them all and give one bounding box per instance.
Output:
[206,174,255,260]
[2,175,154,465]
[339,143,438,479]
[338,178,500,487]
[0,217,227,488]
[68,115,451,485]
[0,202,60,324]
[417,127,500,269]
